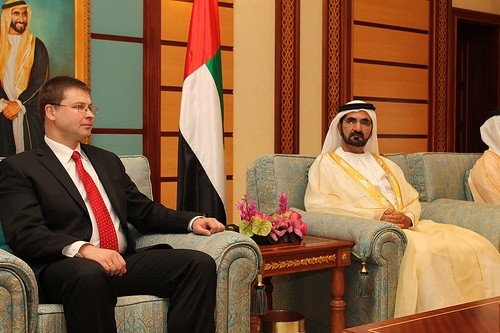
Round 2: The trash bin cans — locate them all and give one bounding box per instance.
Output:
[260,309,307,333]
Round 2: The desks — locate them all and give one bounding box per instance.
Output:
[341,296,500,333]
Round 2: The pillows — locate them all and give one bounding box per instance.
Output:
[465,169,474,202]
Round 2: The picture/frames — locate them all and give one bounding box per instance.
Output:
[0,0,91,155]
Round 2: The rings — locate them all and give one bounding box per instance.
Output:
[109,263,112,266]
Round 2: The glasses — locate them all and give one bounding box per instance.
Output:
[43,102,96,115]
[342,117,373,129]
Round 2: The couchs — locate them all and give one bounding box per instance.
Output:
[407,152,483,204]
[0,155,263,333]
[245,152,500,333]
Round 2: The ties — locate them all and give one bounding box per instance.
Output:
[71,151,119,253]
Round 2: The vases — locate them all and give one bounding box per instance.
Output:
[251,228,304,243]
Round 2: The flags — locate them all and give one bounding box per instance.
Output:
[176,0,227,229]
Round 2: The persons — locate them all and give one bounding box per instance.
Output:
[0,76,226,333]
[468,114,500,204]
[0,0,50,157]
[304,100,500,318]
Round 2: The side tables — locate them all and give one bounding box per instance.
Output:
[251,235,355,333]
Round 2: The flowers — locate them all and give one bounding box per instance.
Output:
[233,192,308,241]
